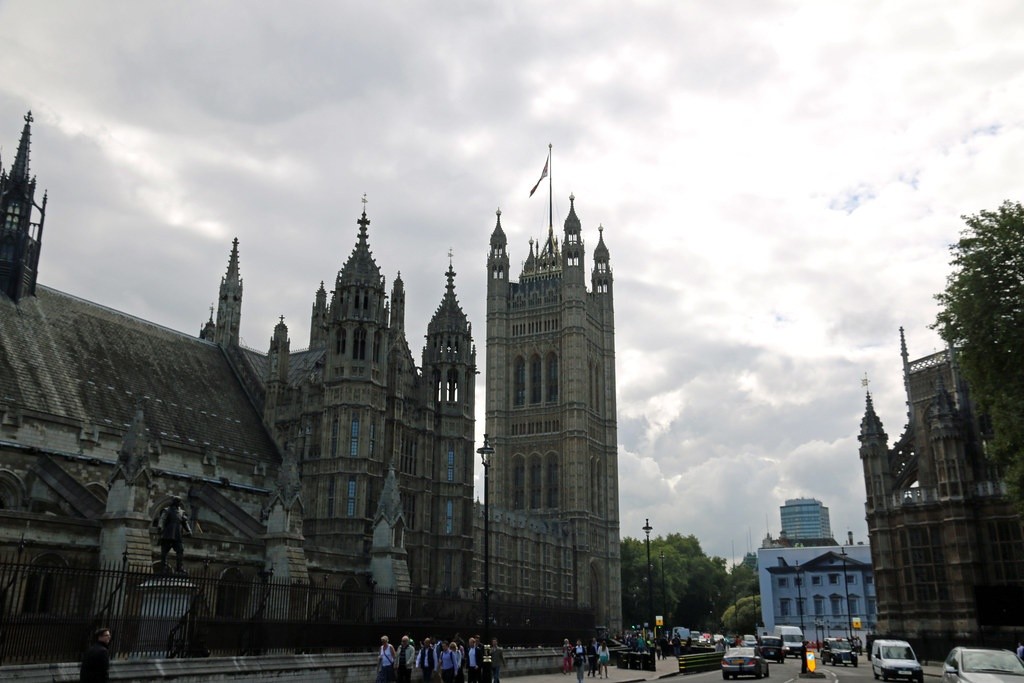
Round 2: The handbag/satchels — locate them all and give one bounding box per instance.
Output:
[392,662,397,672]
[574,658,582,667]
[460,658,465,669]
[597,658,601,665]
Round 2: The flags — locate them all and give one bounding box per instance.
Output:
[529,155,549,197]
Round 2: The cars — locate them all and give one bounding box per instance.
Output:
[741,635,758,648]
[755,636,784,664]
[689,631,738,647]
[721,647,770,680]
[942,646,1024,683]
[820,638,858,667]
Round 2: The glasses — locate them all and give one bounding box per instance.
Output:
[102,633,111,637]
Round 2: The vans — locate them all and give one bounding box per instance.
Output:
[871,640,924,683]
[774,626,808,659]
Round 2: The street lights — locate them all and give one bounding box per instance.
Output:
[641,518,654,632]
[477,433,497,683]
[794,560,808,673]
[840,547,852,639]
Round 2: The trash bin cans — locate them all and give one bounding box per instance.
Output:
[642,653,653,670]
[629,652,641,670]
[616,651,628,669]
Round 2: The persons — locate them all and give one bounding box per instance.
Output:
[735,636,742,647]
[853,637,862,656]
[158,496,192,574]
[616,631,652,652]
[654,632,681,660]
[810,642,815,648]
[685,636,692,653]
[586,637,610,679]
[1016,641,1024,661]
[80,628,112,683]
[376,635,505,683]
[716,638,729,651]
[570,639,586,683]
[562,639,573,674]
